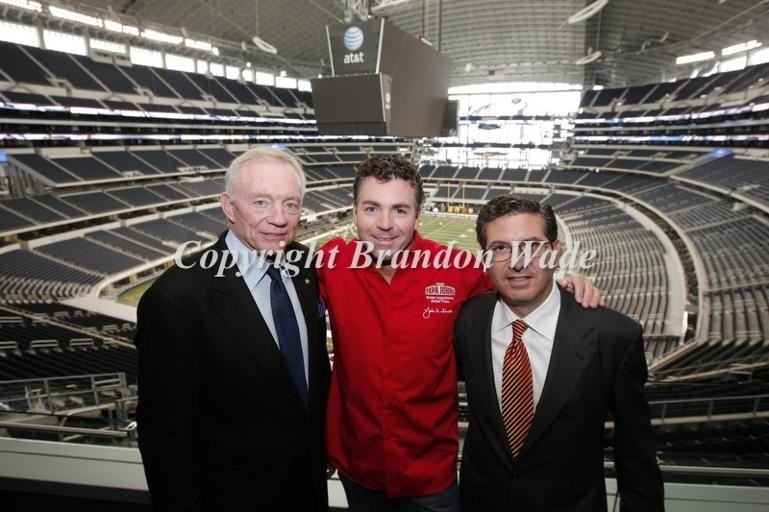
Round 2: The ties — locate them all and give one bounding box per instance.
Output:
[266,265,309,405]
[500,320,535,458]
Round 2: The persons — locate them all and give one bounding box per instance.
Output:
[136,147,664,511]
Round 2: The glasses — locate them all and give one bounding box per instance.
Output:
[483,240,551,263]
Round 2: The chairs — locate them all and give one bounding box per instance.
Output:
[0,41,769,488]
